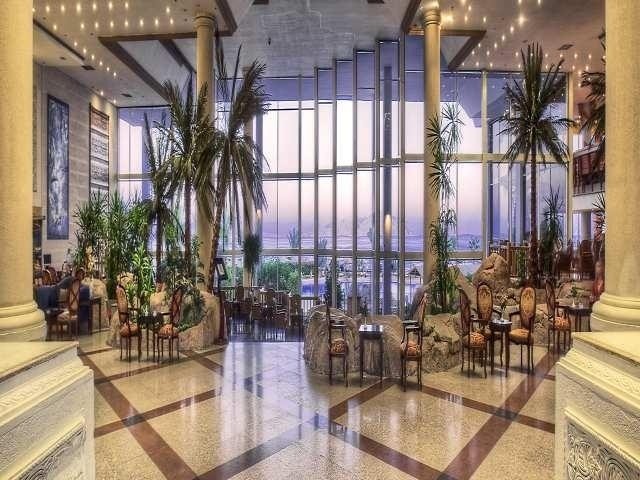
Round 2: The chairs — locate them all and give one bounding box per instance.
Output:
[44,263,185,362]
[230,287,304,338]
[326,280,570,392]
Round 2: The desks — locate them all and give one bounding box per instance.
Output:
[34,283,55,309]
[558,297,592,332]
